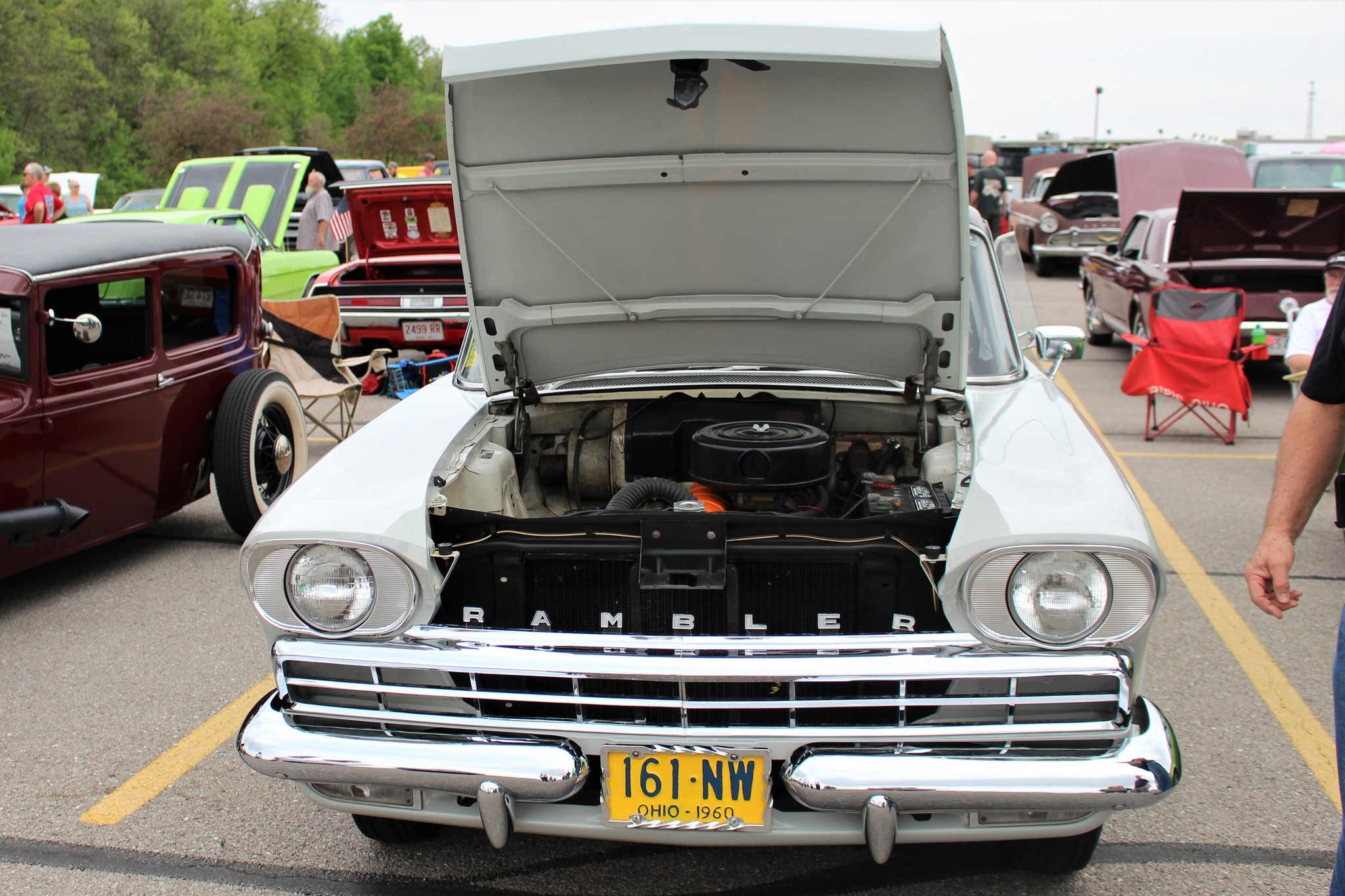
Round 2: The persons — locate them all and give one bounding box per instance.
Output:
[296,172,340,252]
[1246,275,1345,896]
[968,150,1007,240]
[387,154,437,179]
[1284,250,1345,491]
[18,162,93,224]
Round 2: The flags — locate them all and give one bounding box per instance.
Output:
[329,194,354,241]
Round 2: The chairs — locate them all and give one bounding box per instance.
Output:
[1120,286,1276,446]
[261,294,392,443]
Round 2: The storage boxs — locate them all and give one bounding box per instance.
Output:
[1036,326,1086,360]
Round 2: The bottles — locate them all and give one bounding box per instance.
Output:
[1252,324,1266,345]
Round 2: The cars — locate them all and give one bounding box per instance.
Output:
[1008,149,1122,277]
[0,145,470,377]
[1079,139,1345,368]
[236,19,1180,873]
[0,222,307,580]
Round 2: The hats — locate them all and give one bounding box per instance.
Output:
[387,162,397,168]
[423,154,436,160]
[1324,252,1345,271]
[43,165,53,173]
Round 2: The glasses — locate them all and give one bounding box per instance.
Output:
[23,173,30,176]
[71,185,80,188]
